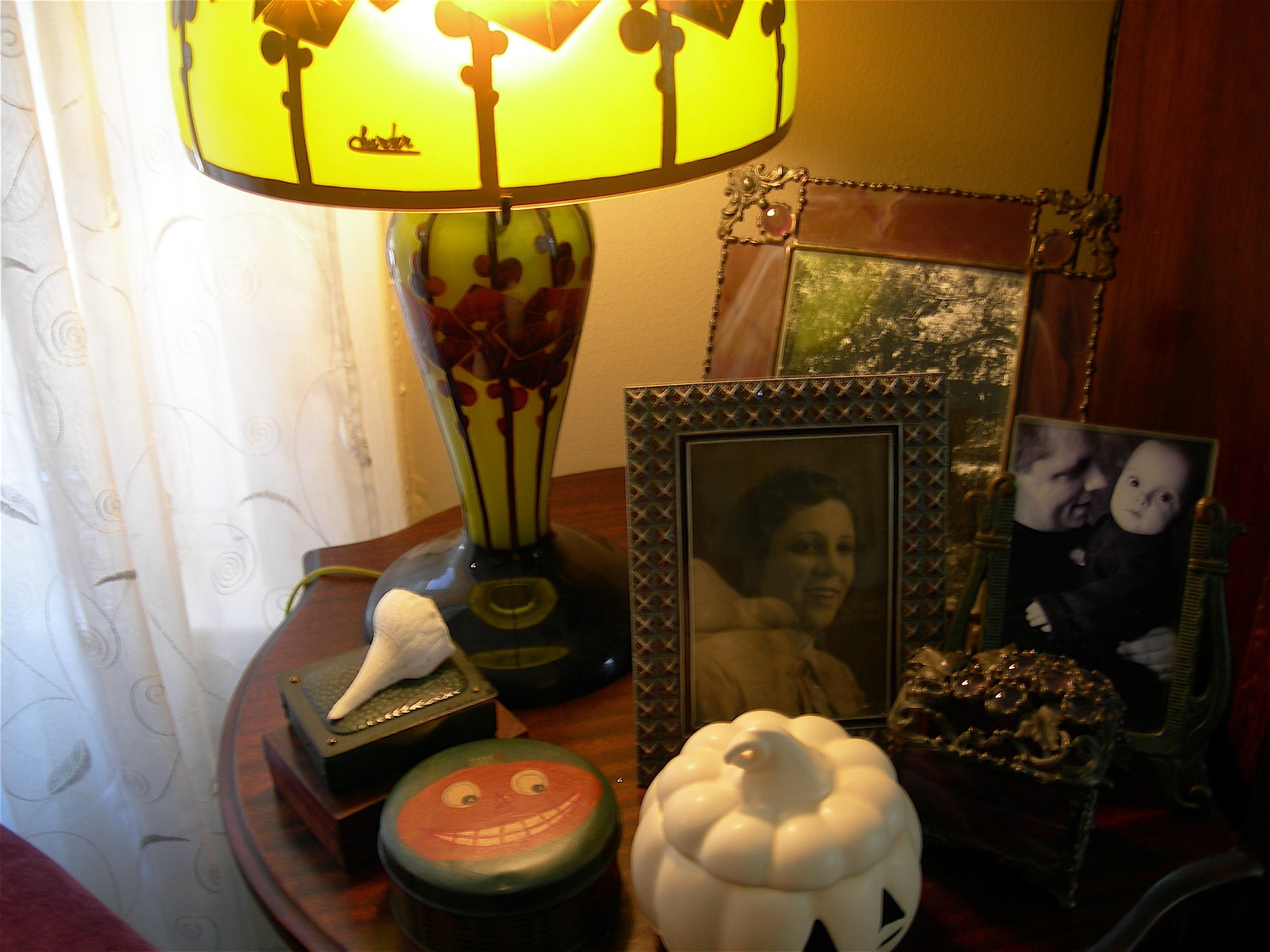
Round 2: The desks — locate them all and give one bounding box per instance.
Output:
[218,467,1270,952]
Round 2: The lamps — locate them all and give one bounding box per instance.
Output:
[167,0,798,708]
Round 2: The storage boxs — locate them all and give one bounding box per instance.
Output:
[261,648,529,863]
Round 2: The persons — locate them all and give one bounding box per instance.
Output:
[693,471,867,720]
[1001,424,1199,732]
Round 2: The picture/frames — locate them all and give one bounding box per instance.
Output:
[619,163,1226,795]
